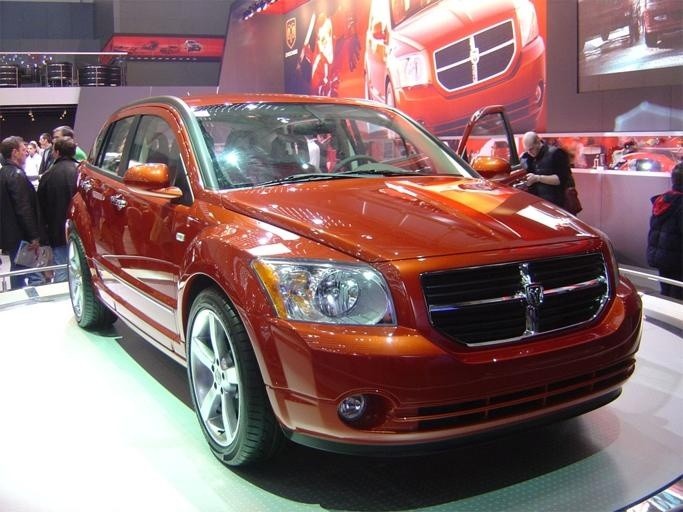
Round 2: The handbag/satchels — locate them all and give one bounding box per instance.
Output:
[562,187,582,215]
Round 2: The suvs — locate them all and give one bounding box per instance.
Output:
[359,0,546,137]
[64,94,645,469]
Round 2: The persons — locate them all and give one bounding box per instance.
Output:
[645,162,683,301]
[519,131,575,212]
[0,126,88,291]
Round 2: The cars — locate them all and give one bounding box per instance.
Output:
[141,40,201,54]
[642,1,682,46]
[578,1,641,51]
[490,141,509,160]
